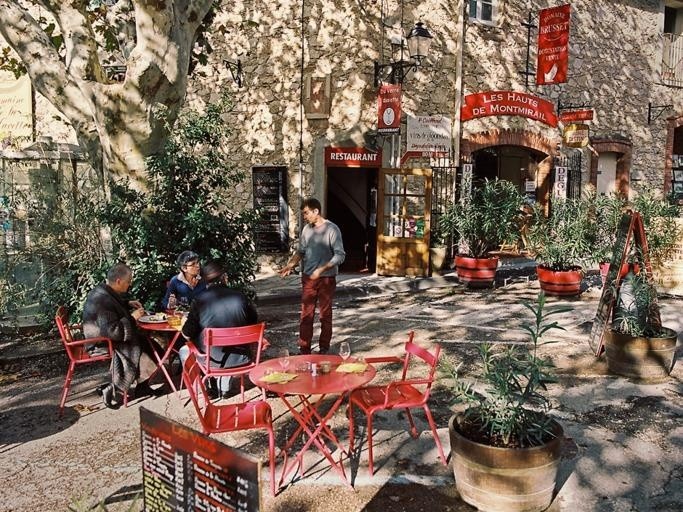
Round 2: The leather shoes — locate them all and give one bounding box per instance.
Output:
[96,385,105,396]
[103,385,114,407]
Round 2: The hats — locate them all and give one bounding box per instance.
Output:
[204,262,229,281]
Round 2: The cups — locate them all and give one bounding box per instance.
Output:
[294,361,306,380]
[278,349,290,380]
[264,366,273,384]
[339,342,351,365]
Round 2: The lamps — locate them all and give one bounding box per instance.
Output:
[374,21,434,88]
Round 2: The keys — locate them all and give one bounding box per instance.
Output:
[81,262,166,409]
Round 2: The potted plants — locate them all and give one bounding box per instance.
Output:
[530,193,598,296]
[439,175,528,286]
[602,244,679,379]
[437,289,576,512]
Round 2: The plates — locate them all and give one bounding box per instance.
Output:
[138,315,169,323]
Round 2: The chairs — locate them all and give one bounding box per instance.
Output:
[183,350,277,499]
[178,321,268,403]
[52,303,128,421]
[344,332,450,475]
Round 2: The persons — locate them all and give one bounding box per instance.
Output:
[279,197,346,355]
[163,251,203,311]
[180,260,259,409]
